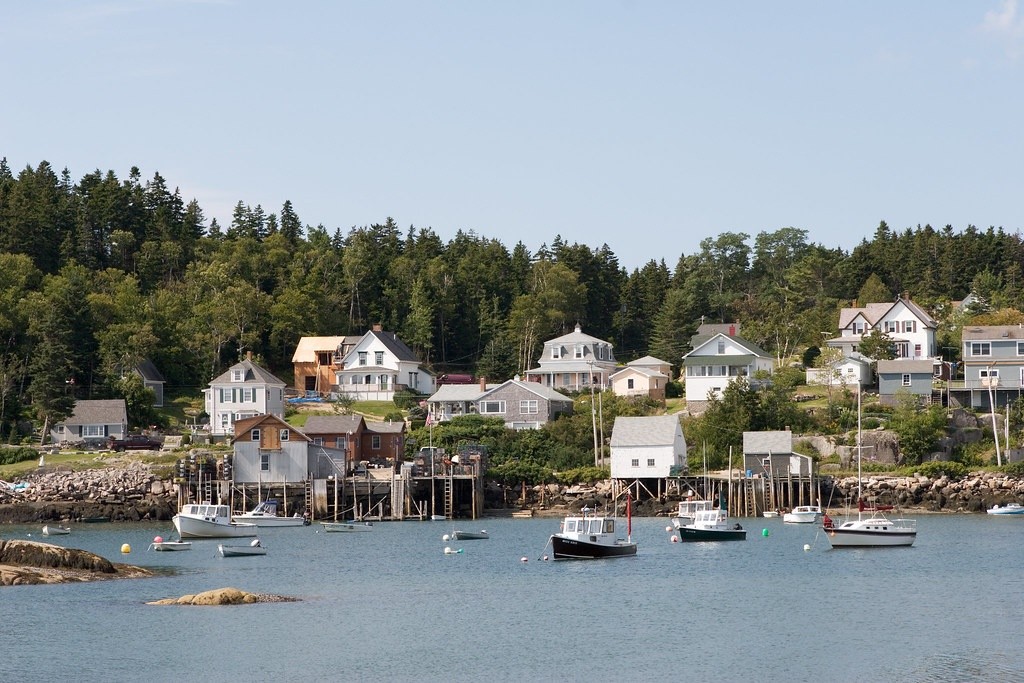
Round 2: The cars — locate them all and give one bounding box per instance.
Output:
[288,395,325,403]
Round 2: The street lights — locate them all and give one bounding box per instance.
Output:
[986,360,1002,467]
[587,360,598,466]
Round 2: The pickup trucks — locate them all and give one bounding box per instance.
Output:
[111,434,161,452]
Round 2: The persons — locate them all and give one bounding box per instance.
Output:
[734,523,742,530]
[823,513,834,527]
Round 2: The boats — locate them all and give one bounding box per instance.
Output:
[152,541,192,551]
[172,501,258,538]
[780,505,821,523]
[454,529,490,540]
[566,485,596,496]
[218,540,267,557]
[986,502,1024,514]
[551,516,639,559]
[672,500,714,529]
[679,509,747,542]
[320,521,373,533]
[762,511,778,518]
[42,524,71,536]
[232,500,304,527]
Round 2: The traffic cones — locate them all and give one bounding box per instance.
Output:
[38,455,46,468]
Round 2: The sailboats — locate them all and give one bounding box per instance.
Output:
[821,380,917,548]
